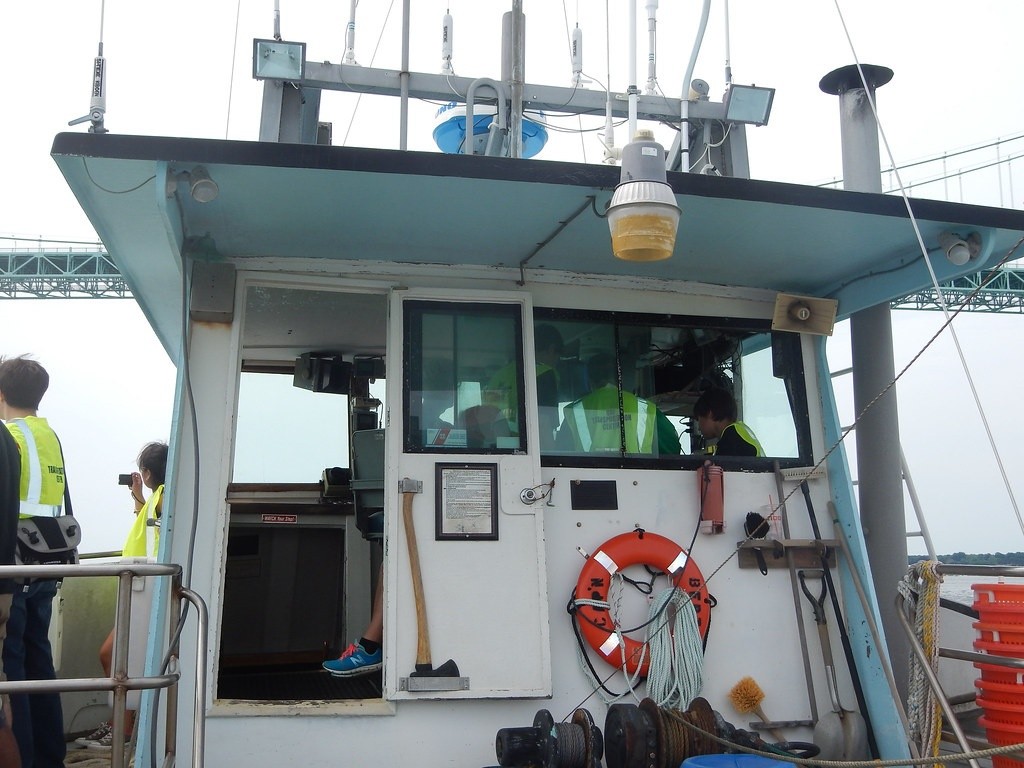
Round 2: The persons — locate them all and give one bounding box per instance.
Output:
[481,324,564,451]
[0,420,24,768]
[322,563,384,674]
[0,353,68,768]
[74,442,170,751]
[556,351,682,455]
[692,387,766,458]
[422,356,454,429]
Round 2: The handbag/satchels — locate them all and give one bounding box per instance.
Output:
[15,515,81,594]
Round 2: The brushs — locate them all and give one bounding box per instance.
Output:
[727,674,800,758]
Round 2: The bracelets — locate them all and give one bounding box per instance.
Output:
[133,508,140,513]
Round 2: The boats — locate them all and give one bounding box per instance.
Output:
[1,0,1023,768]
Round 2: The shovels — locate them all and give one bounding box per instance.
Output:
[799,569,868,768]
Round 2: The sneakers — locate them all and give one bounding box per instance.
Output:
[74,721,111,746]
[87,731,137,749]
[322,640,382,678]
